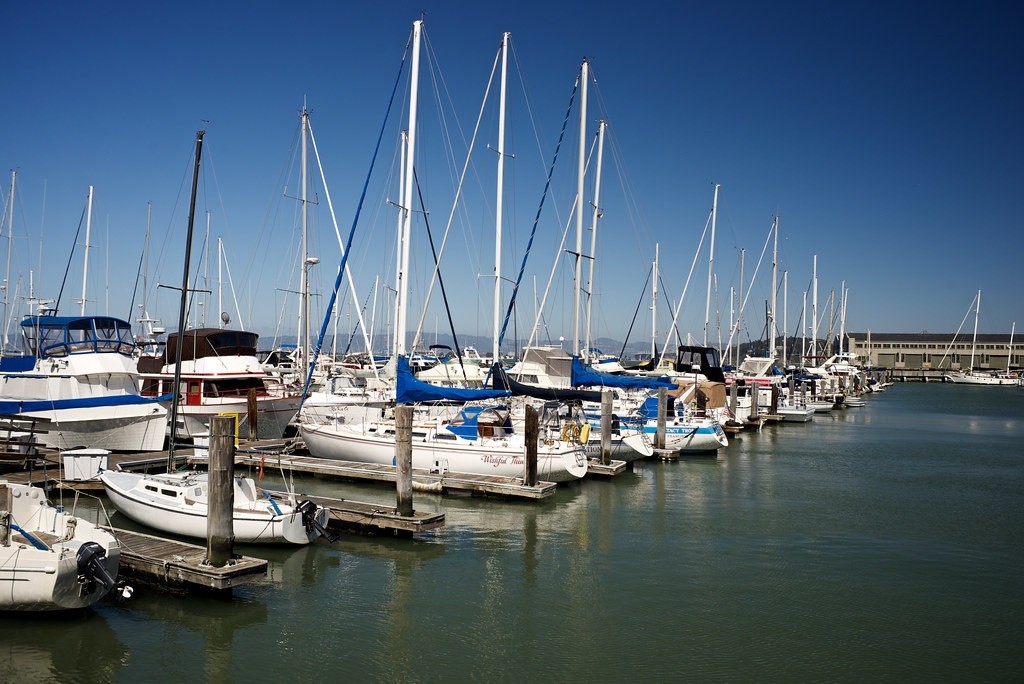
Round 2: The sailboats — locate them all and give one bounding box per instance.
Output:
[0,19,892,484]
[943,289,1024,385]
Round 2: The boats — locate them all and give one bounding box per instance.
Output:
[98,468,340,546]
[1,480,130,614]
